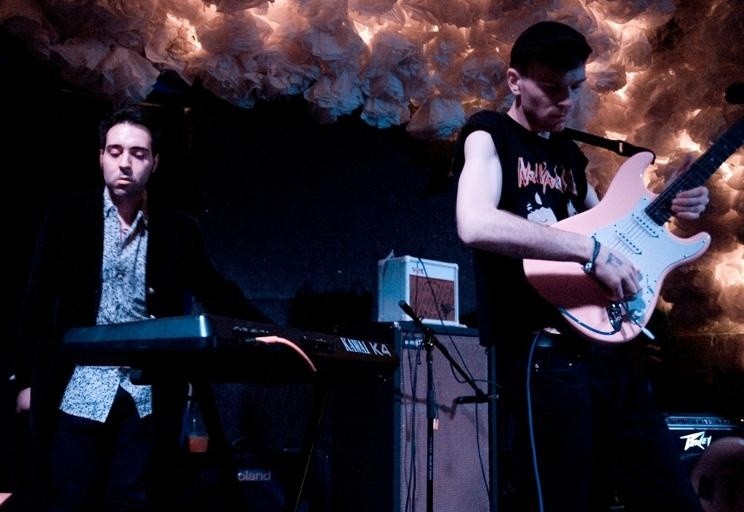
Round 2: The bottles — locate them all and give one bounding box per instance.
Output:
[187,416,210,473]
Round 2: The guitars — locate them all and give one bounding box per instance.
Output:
[523,113,743,343]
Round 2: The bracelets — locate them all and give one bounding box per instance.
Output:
[583,235,601,275]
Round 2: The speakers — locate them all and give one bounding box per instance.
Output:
[369,321,501,511]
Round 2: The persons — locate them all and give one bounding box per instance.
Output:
[10,106,223,512]
[450,21,710,512]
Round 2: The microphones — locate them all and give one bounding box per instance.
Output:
[454,390,503,406]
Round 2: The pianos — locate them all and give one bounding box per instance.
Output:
[62,313,399,385]
